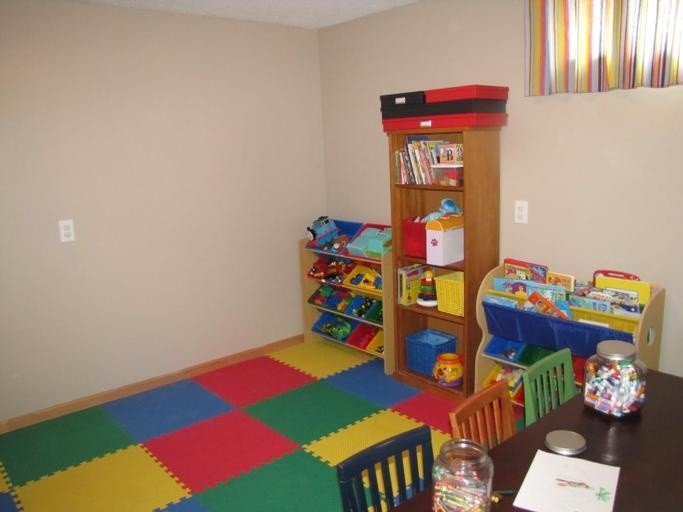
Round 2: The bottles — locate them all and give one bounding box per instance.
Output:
[582,340,648,419]
[433,438,494,507]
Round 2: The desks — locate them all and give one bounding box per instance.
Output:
[393,364,683,511]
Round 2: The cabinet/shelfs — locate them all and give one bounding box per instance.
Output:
[472,260,665,414]
[298,235,394,379]
[387,126,502,404]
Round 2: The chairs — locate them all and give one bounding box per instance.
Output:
[333,348,575,512]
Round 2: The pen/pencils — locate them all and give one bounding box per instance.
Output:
[494,490,517,494]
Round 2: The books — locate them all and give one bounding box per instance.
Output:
[393,134,464,185]
